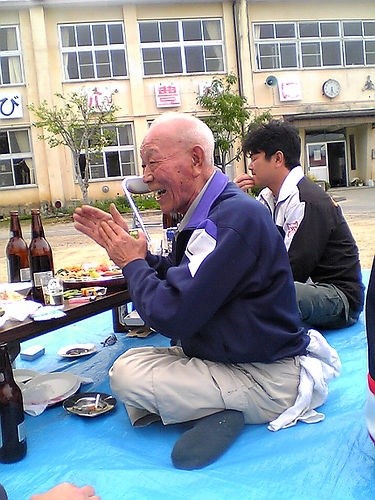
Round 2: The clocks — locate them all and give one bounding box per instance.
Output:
[323,79,340,98]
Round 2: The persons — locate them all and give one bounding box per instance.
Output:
[74,110,342,471]
[232,123,366,328]
[0,482,100,500]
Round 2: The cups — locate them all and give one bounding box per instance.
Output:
[40,275,64,309]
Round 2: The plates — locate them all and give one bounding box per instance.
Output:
[12,369,117,417]
[57,344,97,357]
[59,276,126,288]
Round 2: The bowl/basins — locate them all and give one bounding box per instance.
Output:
[0,282,33,299]
[0,303,8,328]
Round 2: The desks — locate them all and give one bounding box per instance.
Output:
[0,280,131,362]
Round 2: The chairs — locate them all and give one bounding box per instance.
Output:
[122,175,163,243]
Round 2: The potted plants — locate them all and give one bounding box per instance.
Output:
[356,179,364,187]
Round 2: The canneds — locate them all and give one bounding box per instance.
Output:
[166,227,178,253]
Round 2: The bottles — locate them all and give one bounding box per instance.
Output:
[6,211,31,296]
[0,341,27,464]
[28,209,54,302]
